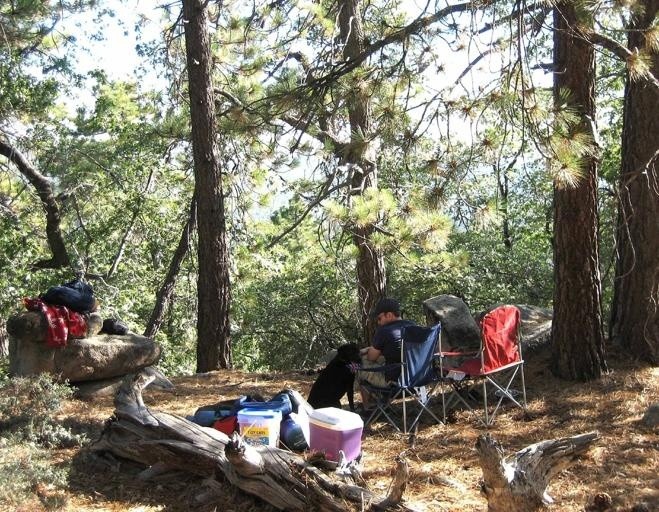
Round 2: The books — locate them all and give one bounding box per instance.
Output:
[311,406,360,425]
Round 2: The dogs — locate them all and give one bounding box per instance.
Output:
[307,342,362,412]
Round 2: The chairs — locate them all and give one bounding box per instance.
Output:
[434,305,527,427]
[346,321,449,435]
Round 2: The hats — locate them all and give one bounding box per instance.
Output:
[374,298,400,314]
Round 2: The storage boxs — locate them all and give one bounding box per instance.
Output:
[237,408,282,447]
[309,407,364,462]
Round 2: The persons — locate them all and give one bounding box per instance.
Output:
[356,298,416,413]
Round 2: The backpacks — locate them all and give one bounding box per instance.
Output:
[39,279,95,312]
